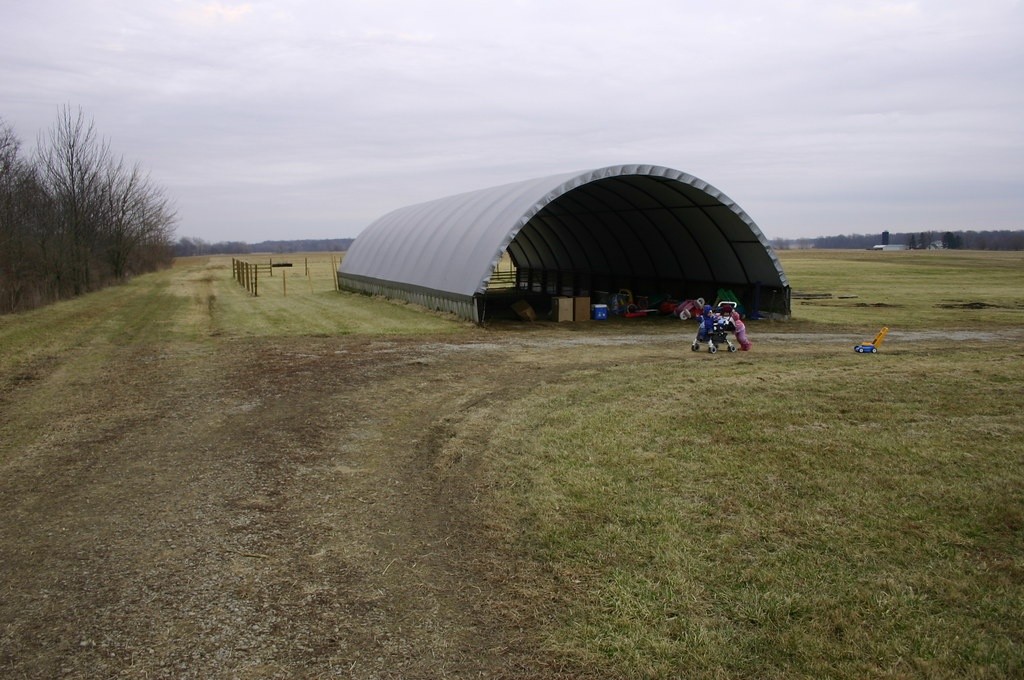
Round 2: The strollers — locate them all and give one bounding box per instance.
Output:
[691,300,738,353]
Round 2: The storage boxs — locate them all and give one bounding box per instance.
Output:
[553,297,608,322]
[511,300,535,320]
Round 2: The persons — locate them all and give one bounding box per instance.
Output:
[733,312,751,350]
